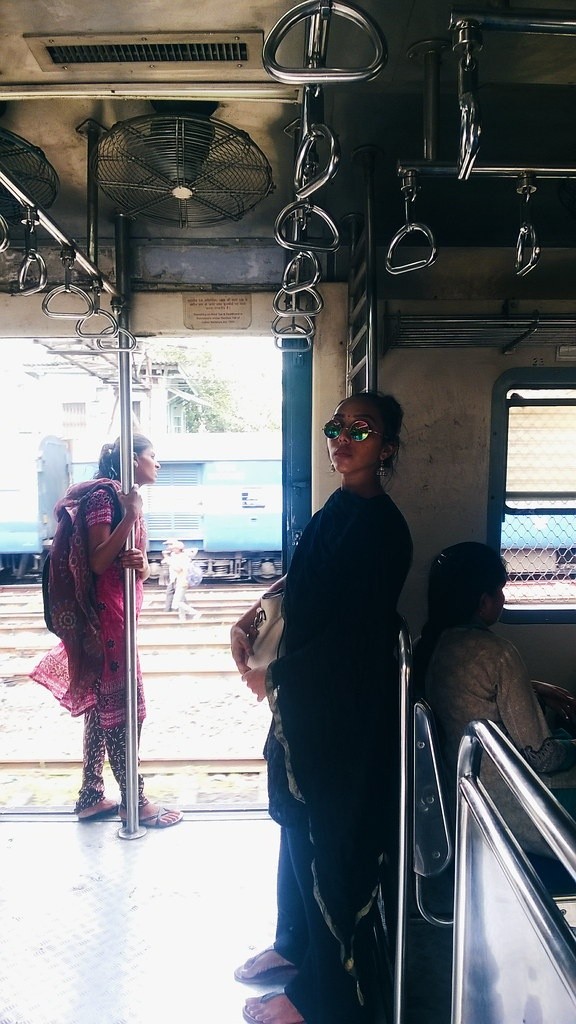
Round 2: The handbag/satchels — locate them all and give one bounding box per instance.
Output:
[246,588,287,670]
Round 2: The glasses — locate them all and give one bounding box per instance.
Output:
[322,420,389,441]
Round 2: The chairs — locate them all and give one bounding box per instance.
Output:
[411,697,576,929]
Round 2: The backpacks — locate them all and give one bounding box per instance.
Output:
[189,562,203,588]
[41,485,125,636]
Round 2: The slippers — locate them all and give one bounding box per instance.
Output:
[122,807,184,829]
[234,946,296,984]
[78,801,121,822]
[243,991,305,1024]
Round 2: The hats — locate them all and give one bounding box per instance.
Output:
[171,542,184,549]
[162,538,178,544]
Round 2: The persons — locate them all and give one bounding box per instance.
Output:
[409,540,576,766]
[41,436,186,829]
[231,394,415,1022]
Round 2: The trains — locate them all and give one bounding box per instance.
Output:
[0,414,576,584]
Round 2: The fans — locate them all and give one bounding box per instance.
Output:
[87,111,275,230]
[0,126,61,227]
